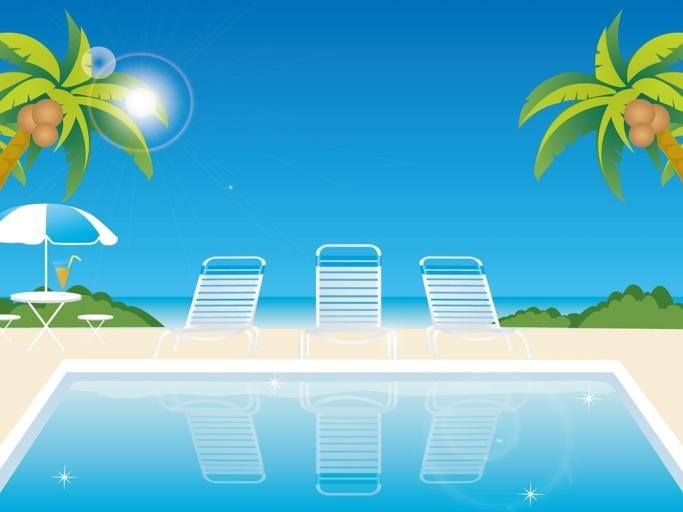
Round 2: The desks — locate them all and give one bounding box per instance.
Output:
[9,291,81,353]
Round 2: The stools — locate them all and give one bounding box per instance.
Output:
[0,314,20,348]
[72,312,115,350]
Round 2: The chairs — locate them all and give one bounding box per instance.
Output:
[416,383,527,486]
[154,381,268,485]
[298,376,397,499]
[159,254,267,345]
[300,240,392,359]
[416,252,529,363]
